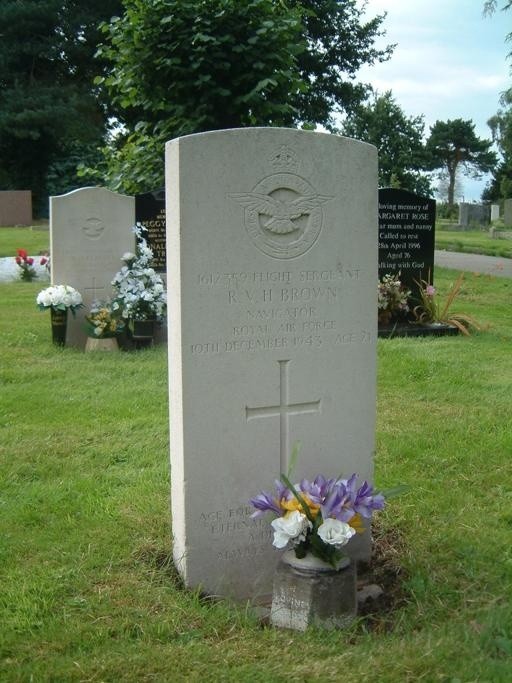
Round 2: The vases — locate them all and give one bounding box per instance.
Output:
[85,335,118,353]
[48,307,68,346]
[270,547,354,631]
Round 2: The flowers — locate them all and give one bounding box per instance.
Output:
[242,439,386,576]
[16,226,167,339]
[373,270,485,338]
